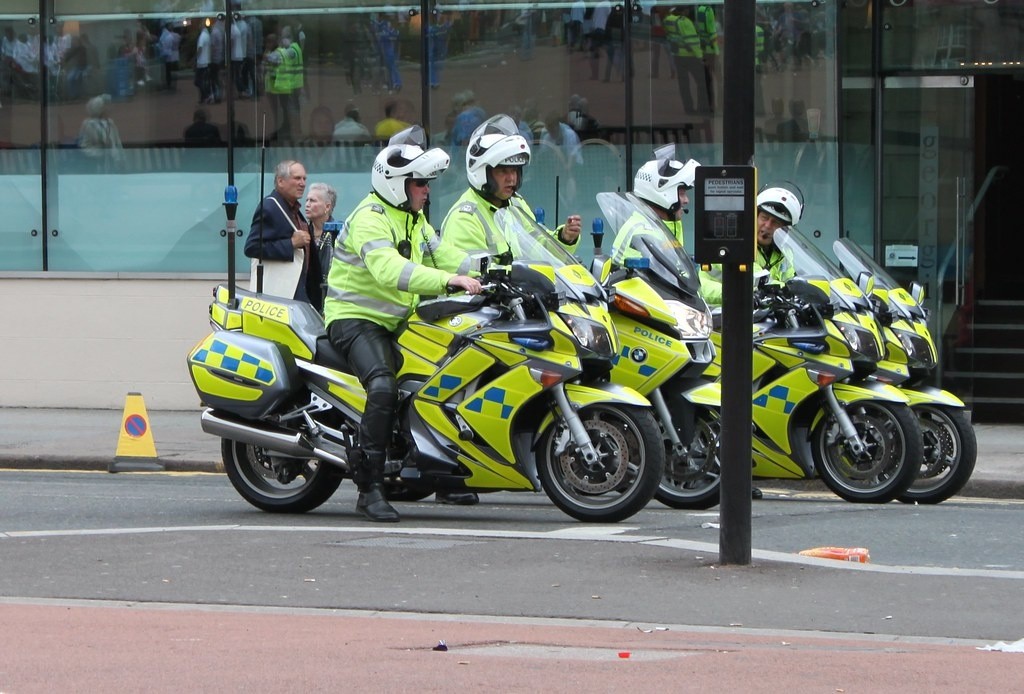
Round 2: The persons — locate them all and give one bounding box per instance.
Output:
[304,182,341,308]
[609,142,724,307]
[0,2,828,170]
[755,178,807,303]
[436,112,581,289]
[244,160,318,312]
[323,124,509,523]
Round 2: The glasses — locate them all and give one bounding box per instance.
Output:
[410,178,430,188]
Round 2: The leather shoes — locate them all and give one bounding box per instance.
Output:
[435,491,479,504]
[355,486,398,522]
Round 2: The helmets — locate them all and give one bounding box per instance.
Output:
[757,186,804,229]
[633,158,702,213]
[465,134,532,193]
[370,142,451,209]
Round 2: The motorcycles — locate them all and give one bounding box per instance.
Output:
[829,235,979,505]
[186,204,666,524]
[701,222,924,504]
[570,191,726,508]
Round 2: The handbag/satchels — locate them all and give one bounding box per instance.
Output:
[249,244,304,300]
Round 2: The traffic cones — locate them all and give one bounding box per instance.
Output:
[107,389,166,473]
